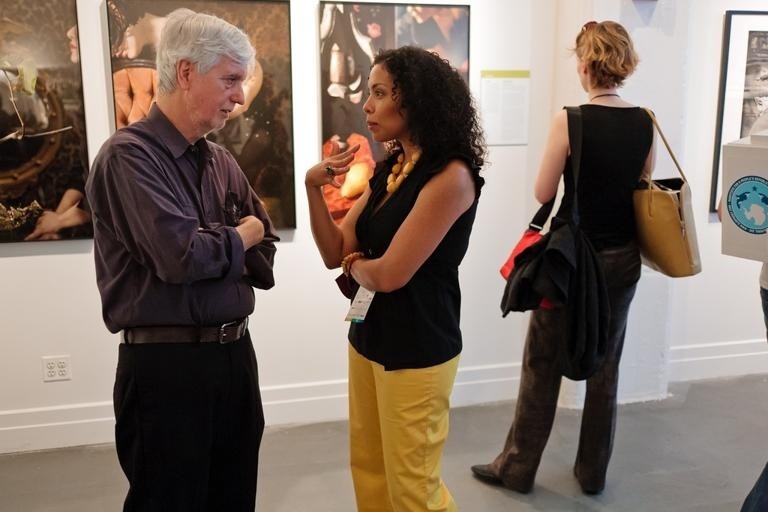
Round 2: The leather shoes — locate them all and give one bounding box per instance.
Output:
[472,465,528,492]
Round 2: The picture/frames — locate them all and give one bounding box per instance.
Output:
[709,10,768,213]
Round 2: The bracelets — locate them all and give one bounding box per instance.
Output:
[341,251,365,277]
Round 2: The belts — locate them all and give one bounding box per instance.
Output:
[120,317,249,344]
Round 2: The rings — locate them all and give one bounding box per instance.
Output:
[326,165,335,178]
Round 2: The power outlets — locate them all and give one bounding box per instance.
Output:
[42,354,72,382]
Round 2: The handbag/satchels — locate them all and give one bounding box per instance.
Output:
[633,178,701,277]
[500,230,554,309]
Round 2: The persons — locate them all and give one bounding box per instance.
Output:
[84,9,280,512]
[471,20,655,495]
[718,113,768,512]
[306,46,484,512]
[25,0,126,241]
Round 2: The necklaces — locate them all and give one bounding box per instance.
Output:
[386,149,422,193]
[590,94,619,102]
